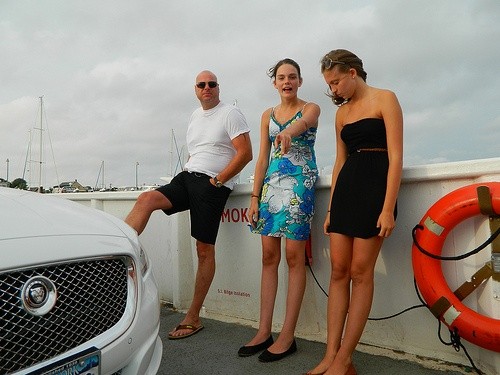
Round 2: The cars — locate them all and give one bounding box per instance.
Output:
[0,185,164,375]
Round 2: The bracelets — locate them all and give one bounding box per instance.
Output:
[251,195,259,198]
[328,210,330,212]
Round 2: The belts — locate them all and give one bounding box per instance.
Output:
[191,172,211,182]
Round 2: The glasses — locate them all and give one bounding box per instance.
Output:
[323,57,345,69]
[196,81,217,88]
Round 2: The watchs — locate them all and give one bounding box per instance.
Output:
[214,177,222,188]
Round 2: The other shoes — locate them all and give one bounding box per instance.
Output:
[238,334,274,357]
[258,338,298,361]
[308,361,357,375]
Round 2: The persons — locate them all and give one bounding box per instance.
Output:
[303,48,404,375]
[236,58,322,364]
[123,70,254,339]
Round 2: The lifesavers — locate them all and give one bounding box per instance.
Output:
[411,181,500,353]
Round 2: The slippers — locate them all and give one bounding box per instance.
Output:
[169,322,204,338]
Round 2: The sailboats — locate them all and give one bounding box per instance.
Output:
[19,96,62,194]
[159,129,187,182]
[91,160,115,192]
